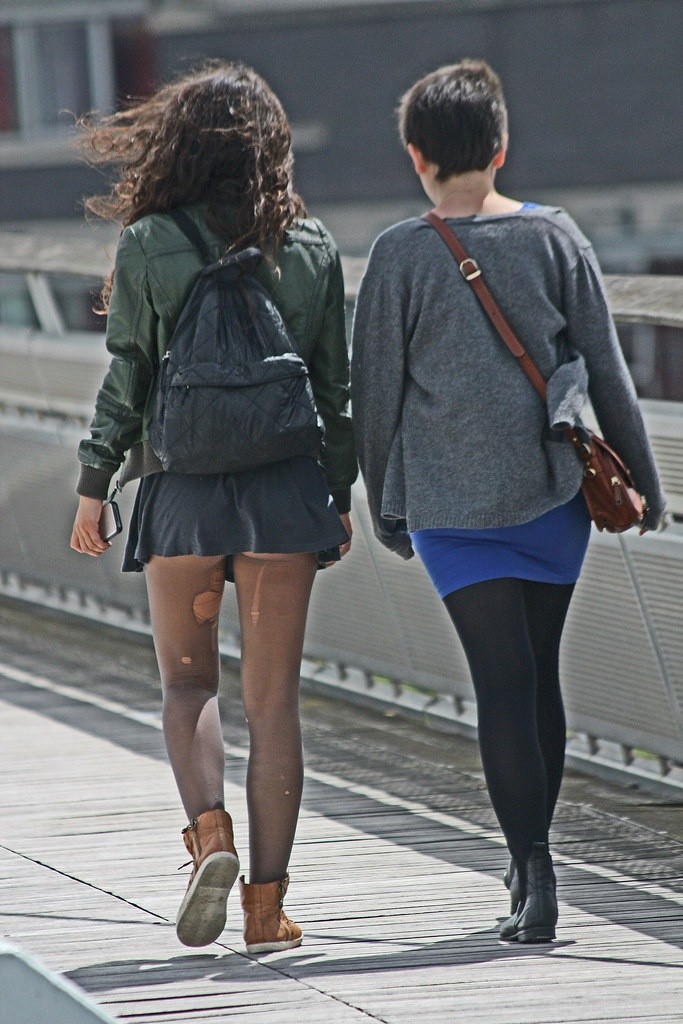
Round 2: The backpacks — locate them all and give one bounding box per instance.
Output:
[149,209,326,472]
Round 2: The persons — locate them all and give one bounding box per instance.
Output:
[351,58,667,943]
[69,58,358,953]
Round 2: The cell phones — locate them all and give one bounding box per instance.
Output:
[99,502,122,542]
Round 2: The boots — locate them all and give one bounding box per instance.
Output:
[239,874,303,954]
[499,841,558,944]
[175,809,239,947]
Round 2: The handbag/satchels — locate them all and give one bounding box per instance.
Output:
[582,435,646,533]
